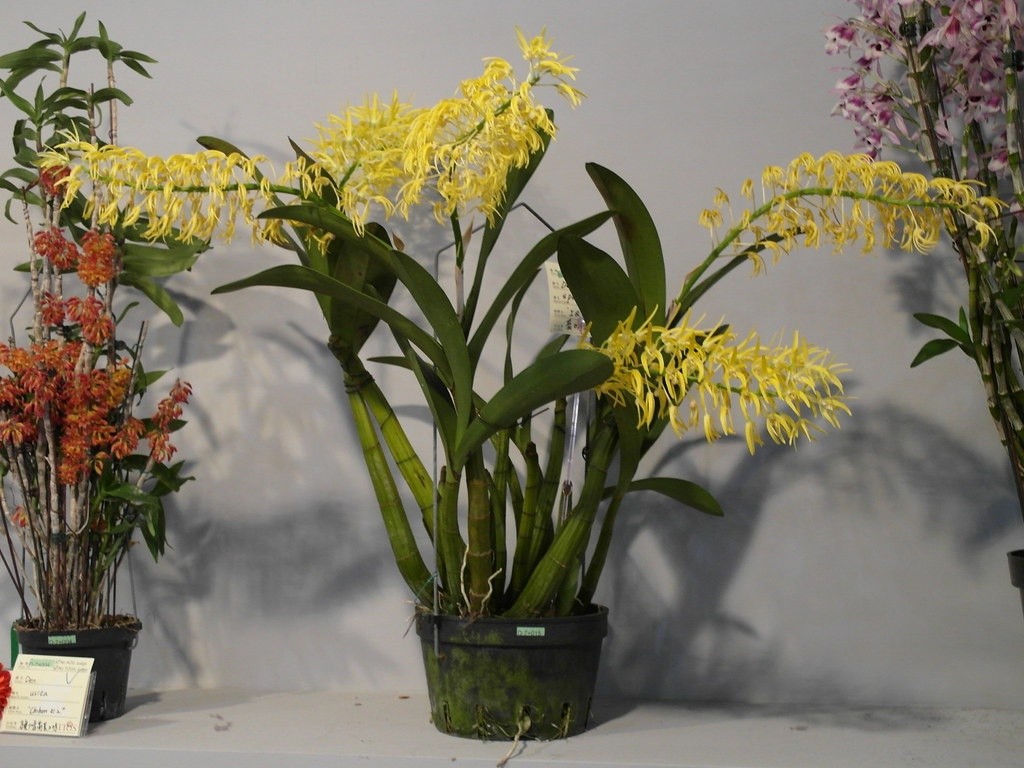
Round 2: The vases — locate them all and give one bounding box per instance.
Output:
[14,614,142,721]
[1006,549,1024,614]
[33,28,1009,617]
[413,599,609,741]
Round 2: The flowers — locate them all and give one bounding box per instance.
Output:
[0,6,194,623]
[825,1,1023,507]
